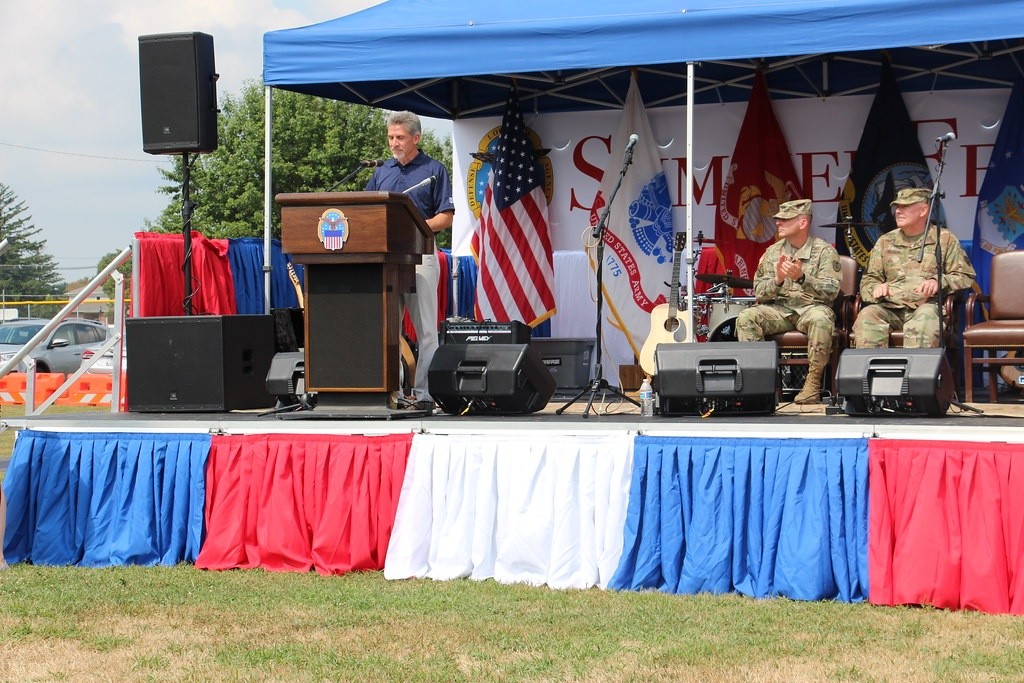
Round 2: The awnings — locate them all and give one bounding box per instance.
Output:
[263,0,1024,314]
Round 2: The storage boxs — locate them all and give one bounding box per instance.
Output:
[531,338,596,388]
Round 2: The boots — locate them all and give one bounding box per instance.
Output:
[795,369,823,404]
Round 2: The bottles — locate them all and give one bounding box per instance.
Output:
[639,379,653,417]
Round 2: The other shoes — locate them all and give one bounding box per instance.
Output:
[390,403,406,419]
[415,399,433,416]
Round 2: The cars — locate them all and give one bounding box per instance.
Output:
[80,333,127,374]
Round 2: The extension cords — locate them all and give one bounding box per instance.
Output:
[826,406,845,415]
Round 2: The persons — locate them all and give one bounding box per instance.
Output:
[852,188,977,349]
[365,111,455,408]
[736,199,843,404]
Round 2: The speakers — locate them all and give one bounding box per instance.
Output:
[127,314,275,414]
[427,321,597,417]
[655,339,779,419]
[139,32,218,153]
[834,348,954,418]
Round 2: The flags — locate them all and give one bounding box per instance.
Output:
[586,72,682,381]
[474,77,558,329]
[835,55,946,292]
[953,63,1024,388]
[713,66,805,298]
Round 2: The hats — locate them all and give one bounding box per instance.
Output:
[890,188,933,207]
[772,199,813,219]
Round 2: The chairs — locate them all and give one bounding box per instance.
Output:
[849,291,964,400]
[764,254,859,404]
[962,249,1024,404]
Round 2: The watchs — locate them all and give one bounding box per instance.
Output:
[797,274,805,285]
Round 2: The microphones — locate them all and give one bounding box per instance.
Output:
[402,175,436,195]
[361,160,384,167]
[626,134,639,153]
[936,132,955,142]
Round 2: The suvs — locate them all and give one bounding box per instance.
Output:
[0,317,110,374]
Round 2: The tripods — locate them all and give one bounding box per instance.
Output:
[256,392,314,417]
[556,149,642,418]
